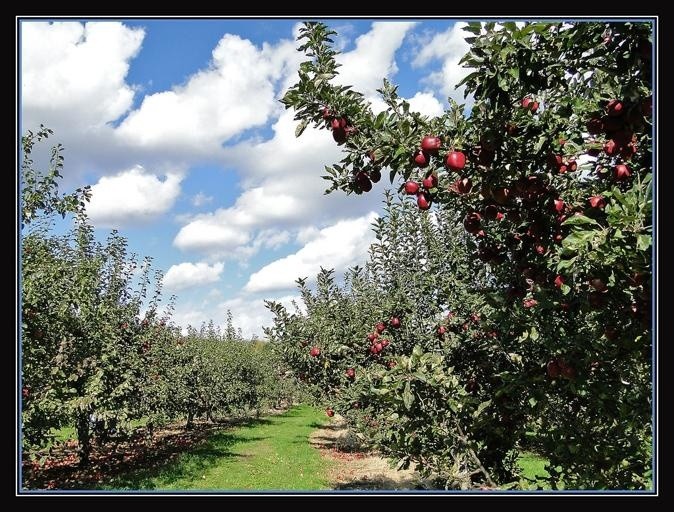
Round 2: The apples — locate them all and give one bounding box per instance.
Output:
[310,97,653,418]
